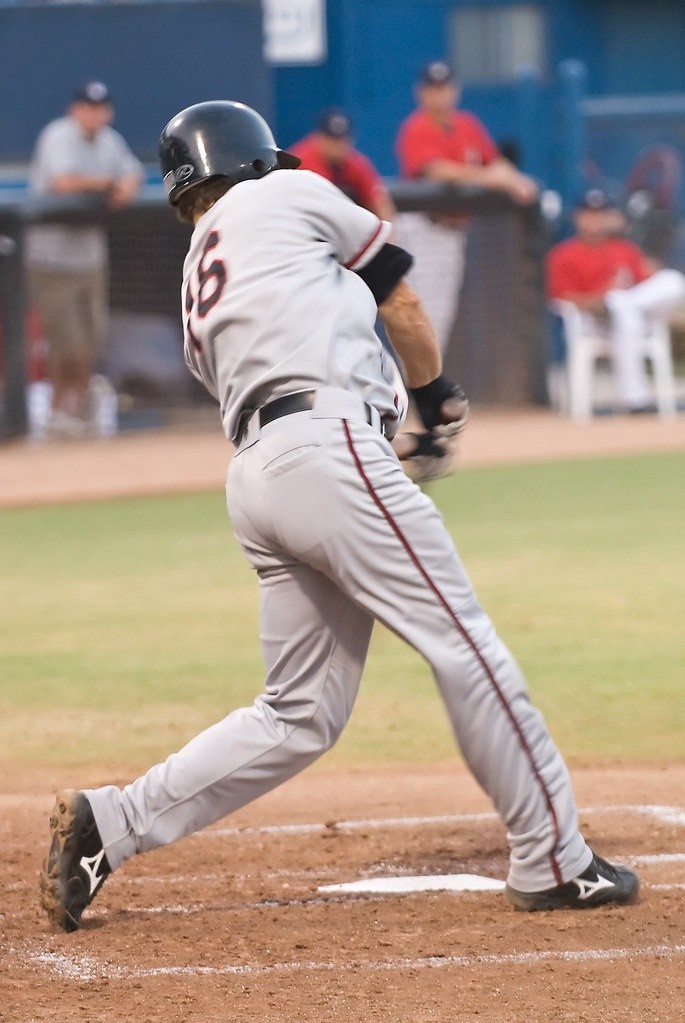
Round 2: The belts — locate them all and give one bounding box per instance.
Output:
[234,390,386,447]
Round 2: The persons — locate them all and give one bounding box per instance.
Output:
[39,101,639,930]
[28,80,145,435]
[544,182,685,414]
[390,61,536,372]
[286,112,397,224]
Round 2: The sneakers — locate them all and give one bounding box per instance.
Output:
[504,849,639,912]
[39,789,112,935]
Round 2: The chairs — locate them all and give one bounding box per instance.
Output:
[550,300,675,420]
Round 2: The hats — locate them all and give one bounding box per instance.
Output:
[322,114,350,137]
[422,62,451,85]
[76,81,112,104]
[575,188,615,210]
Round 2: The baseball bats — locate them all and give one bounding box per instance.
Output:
[438,397,465,421]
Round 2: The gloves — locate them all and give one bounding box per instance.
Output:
[400,432,459,483]
[410,374,470,437]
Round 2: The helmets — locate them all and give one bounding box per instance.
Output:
[158,99,302,206]
